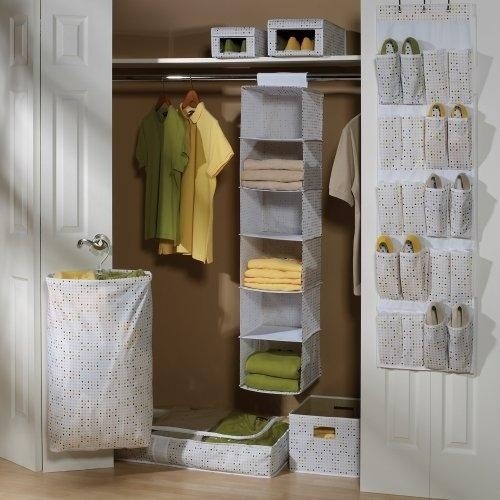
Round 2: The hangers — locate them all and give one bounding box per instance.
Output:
[183,72,200,109]
[156,71,171,111]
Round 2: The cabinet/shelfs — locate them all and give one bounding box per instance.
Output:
[237,85,324,395]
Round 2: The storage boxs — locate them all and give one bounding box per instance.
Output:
[268,18,345,56]
[289,395,360,478]
[211,26,268,58]
